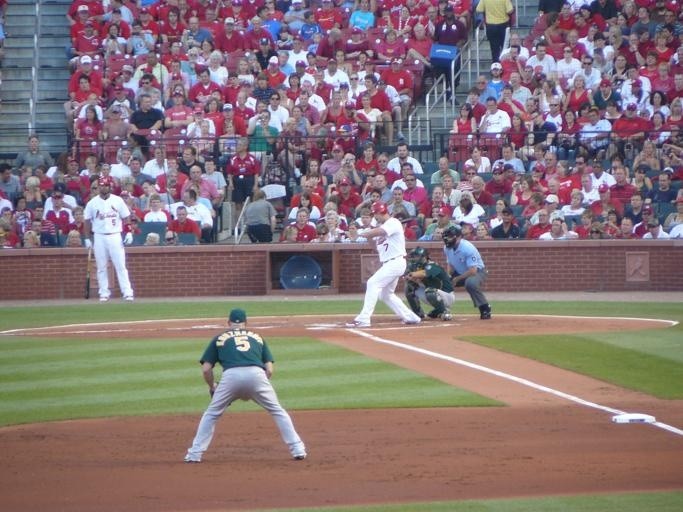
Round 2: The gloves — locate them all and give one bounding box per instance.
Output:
[124,232,133,245]
[84,238,92,248]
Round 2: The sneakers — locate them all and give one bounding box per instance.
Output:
[428,309,451,320]
[184,454,201,461]
[346,320,370,327]
[480,304,491,319]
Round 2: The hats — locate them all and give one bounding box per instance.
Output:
[78,5,182,90]
[491,63,502,70]
[493,164,609,204]
[600,79,641,110]
[229,309,245,322]
[439,207,450,216]
[340,178,351,185]
[225,16,401,112]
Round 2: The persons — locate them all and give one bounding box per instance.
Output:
[182,307,307,464]
[83,176,134,304]
[0,2,682,245]
[341,201,425,328]
[427,226,491,322]
[403,246,455,323]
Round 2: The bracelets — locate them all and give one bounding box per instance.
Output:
[124,223,131,232]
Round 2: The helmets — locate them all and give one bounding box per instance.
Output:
[370,202,387,214]
[442,224,461,247]
[408,247,427,270]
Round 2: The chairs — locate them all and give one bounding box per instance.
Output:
[0,154,681,245]
[66,1,682,154]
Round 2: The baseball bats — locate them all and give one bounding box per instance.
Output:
[401,217,412,224]
[85,248,91,299]
